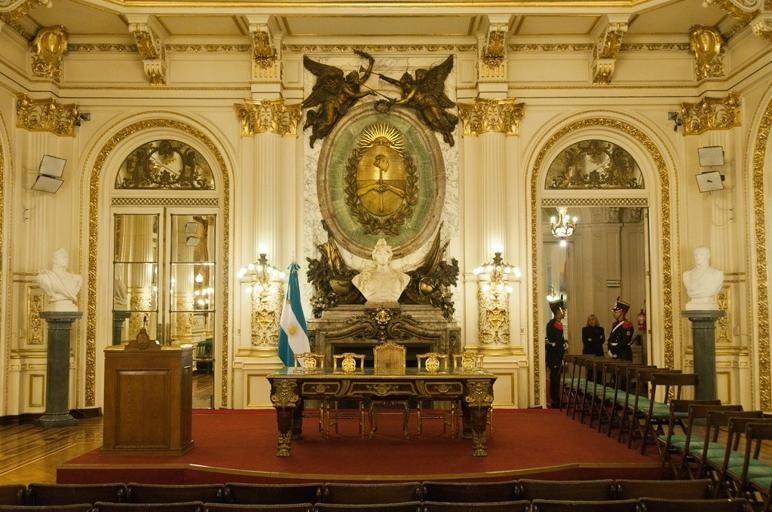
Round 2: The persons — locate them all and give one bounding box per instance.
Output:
[545,300,569,408]
[607,299,634,361]
[350,238,411,302]
[379,68,457,147]
[581,314,606,384]
[682,246,725,299]
[35,249,83,302]
[302,57,378,150]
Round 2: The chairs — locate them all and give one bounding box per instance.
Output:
[705,418,769,497]
[559,353,654,444]
[654,400,722,466]
[638,372,696,458]
[726,421,772,499]
[689,410,763,479]
[416,353,456,439]
[369,342,411,438]
[453,351,493,438]
[324,353,364,440]
[670,405,745,481]
[0,477,747,512]
[290,353,321,434]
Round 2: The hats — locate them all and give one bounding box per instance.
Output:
[549,294,568,309]
[610,296,630,312]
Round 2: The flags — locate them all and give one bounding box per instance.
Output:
[277,262,312,367]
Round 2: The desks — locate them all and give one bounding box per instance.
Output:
[266,368,497,458]
[102,344,195,459]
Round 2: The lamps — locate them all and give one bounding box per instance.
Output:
[697,146,725,167]
[695,171,724,195]
[20,154,67,178]
[20,171,65,194]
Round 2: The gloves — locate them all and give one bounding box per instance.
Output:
[607,349,613,358]
[563,342,569,350]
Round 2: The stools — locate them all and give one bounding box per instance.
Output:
[752,477,772,512]
